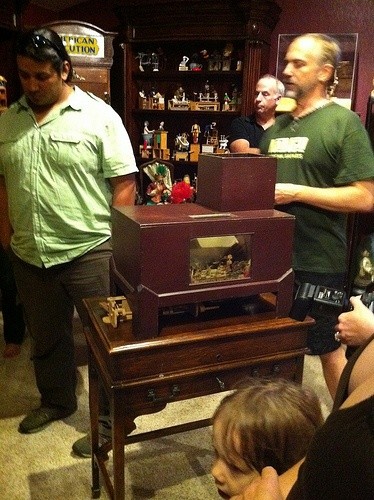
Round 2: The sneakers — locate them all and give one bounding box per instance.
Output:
[72,424,114,457]
[20,403,69,434]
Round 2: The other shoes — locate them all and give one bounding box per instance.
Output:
[2,343,20,358]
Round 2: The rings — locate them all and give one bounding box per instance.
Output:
[334,331,341,342]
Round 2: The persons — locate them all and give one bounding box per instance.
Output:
[230,331,374,500]
[204,122,217,137]
[258,33,374,402]
[144,121,155,135]
[191,124,201,144]
[228,74,286,155]
[224,92,232,105]
[209,376,325,500]
[146,166,185,206]
[0,250,26,359]
[334,295,374,347]
[0,27,139,458]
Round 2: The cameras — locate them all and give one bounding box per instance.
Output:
[288,282,374,326]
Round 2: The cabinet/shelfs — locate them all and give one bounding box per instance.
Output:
[118,0,283,185]
[42,20,119,105]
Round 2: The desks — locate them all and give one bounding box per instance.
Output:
[80,296,316,500]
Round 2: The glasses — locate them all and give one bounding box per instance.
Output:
[20,34,61,53]
